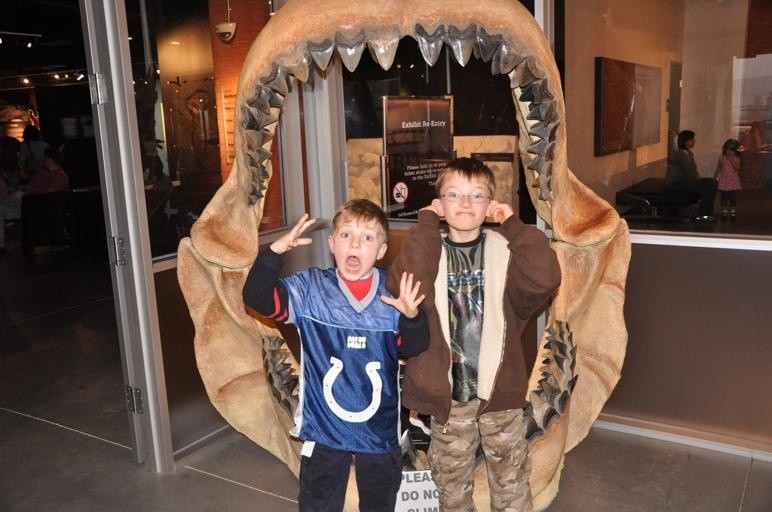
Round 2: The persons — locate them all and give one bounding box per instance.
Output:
[712,139,742,219]
[3,124,24,166]
[384,153,560,511]
[15,124,51,169]
[10,147,70,216]
[668,131,717,226]
[242,198,430,512]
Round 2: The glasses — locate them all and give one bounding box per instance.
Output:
[437,188,492,205]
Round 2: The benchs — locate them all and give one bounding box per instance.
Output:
[616,175,697,224]
